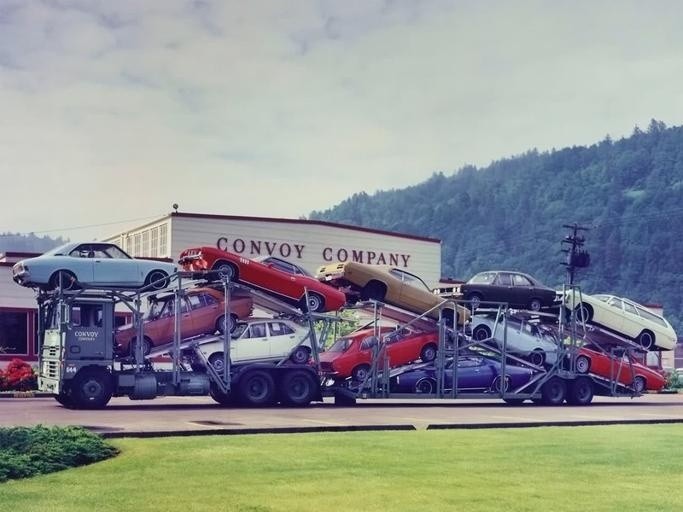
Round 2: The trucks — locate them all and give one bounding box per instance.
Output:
[35,291,649,411]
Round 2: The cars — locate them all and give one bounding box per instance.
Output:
[9,235,177,296]
[177,245,346,317]
[110,284,253,362]
[180,317,320,375]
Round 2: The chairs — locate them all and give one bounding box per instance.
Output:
[80,308,99,327]
[248,326,260,339]
[85,251,95,257]
[365,335,382,350]
